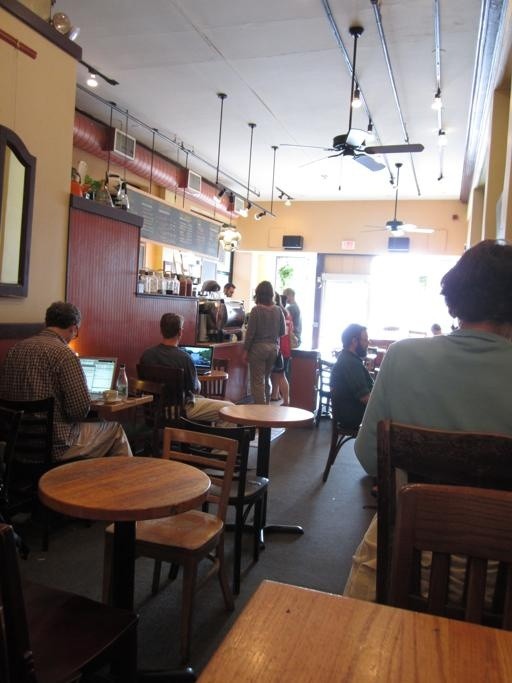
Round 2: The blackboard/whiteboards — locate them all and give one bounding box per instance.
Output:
[125,183,222,261]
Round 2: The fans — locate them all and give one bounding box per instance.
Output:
[278,23,424,173]
[359,160,437,232]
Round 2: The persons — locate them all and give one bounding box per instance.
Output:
[280,294,297,376]
[330,322,378,497]
[430,323,442,336]
[243,280,280,404]
[270,291,290,406]
[139,311,238,454]
[353,237,511,478]
[283,287,302,379]
[223,283,235,298]
[0,300,132,457]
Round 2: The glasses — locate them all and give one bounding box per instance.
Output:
[72,326,79,338]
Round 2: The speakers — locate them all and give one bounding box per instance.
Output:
[283,235,303,250]
[387,236,410,251]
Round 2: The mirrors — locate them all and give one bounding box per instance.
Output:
[1,121,38,299]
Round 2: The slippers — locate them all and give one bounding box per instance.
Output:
[271,397,281,401]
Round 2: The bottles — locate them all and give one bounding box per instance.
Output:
[115,362,128,399]
[139,268,192,295]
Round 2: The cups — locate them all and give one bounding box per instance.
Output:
[102,390,117,399]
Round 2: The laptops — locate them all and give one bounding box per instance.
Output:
[178,344,214,375]
[78,356,119,402]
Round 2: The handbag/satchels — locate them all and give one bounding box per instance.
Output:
[273,354,284,372]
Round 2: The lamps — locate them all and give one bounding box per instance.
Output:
[428,0,447,182]
[214,91,278,220]
[342,151,355,159]
[276,185,295,207]
[80,55,118,92]
[217,211,243,254]
[322,0,397,190]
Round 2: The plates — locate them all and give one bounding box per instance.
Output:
[102,398,121,403]
